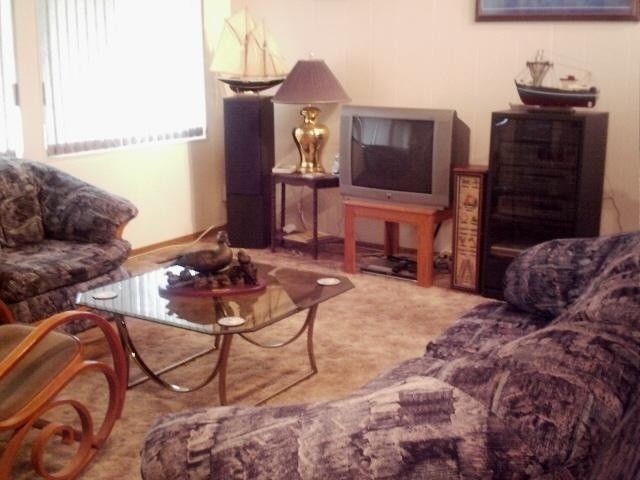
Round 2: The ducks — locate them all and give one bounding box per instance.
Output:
[170,230,233,277]
[163,266,194,285]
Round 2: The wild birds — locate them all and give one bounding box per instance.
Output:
[235,250,251,264]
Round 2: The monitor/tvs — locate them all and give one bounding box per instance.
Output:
[338,103,471,209]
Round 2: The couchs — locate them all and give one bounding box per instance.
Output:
[141,222,640,480]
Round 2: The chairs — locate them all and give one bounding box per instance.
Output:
[0,300,128,480]
[0,158,137,352]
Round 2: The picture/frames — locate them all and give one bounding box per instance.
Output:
[476,0,640,20]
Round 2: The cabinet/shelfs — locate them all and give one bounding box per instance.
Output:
[481,109,609,299]
[223,95,274,248]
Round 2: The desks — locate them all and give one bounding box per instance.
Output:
[342,200,453,288]
[270,172,340,260]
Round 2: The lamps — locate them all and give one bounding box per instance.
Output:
[270,59,350,175]
